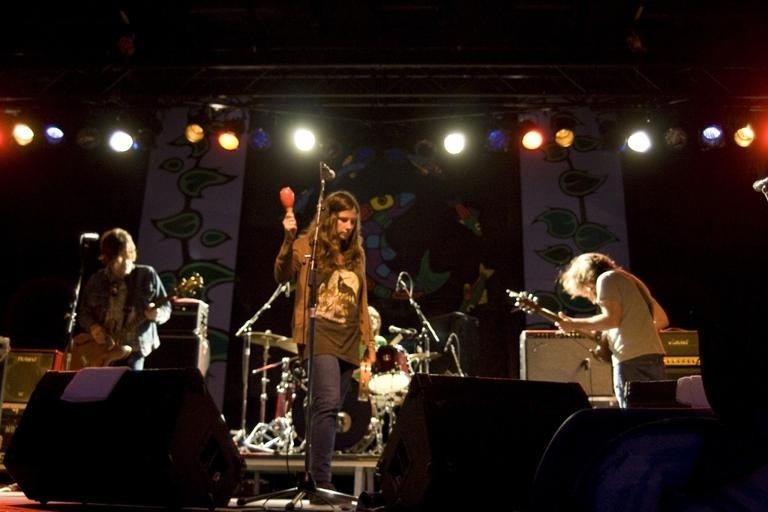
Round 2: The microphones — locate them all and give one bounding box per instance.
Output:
[82,232,99,240]
[320,160,336,180]
[388,325,411,335]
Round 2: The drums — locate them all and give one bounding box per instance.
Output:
[364,344,413,373]
[366,373,413,409]
[292,372,383,452]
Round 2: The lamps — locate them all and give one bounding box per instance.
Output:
[0,89,767,161]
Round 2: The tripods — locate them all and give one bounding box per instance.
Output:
[229,334,251,442]
[240,352,277,445]
[236,316,359,512]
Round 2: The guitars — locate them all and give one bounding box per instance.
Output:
[61,274,205,372]
[504,289,613,363]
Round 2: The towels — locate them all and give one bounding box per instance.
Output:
[60,366,129,402]
[676,376,711,410]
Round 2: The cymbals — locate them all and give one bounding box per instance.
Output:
[277,337,300,353]
[240,332,286,347]
[409,352,441,360]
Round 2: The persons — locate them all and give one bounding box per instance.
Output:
[272,187,374,505]
[552,251,670,409]
[78,228,174,369]
[352,305,385,383]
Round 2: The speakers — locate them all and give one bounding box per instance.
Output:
[2,367,248,508]
[1,348,65,401]
[373,373,591,512]
